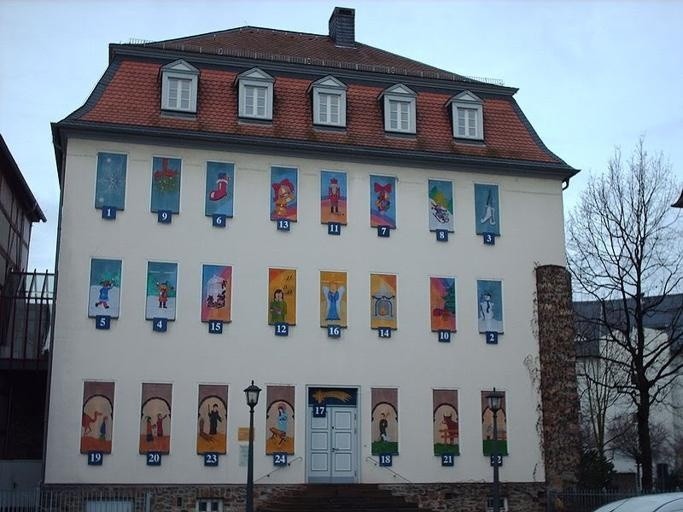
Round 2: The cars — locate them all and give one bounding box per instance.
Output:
[591,490,683,512]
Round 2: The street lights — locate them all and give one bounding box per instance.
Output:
[241,378,264,512]
[486,385,504,510]
